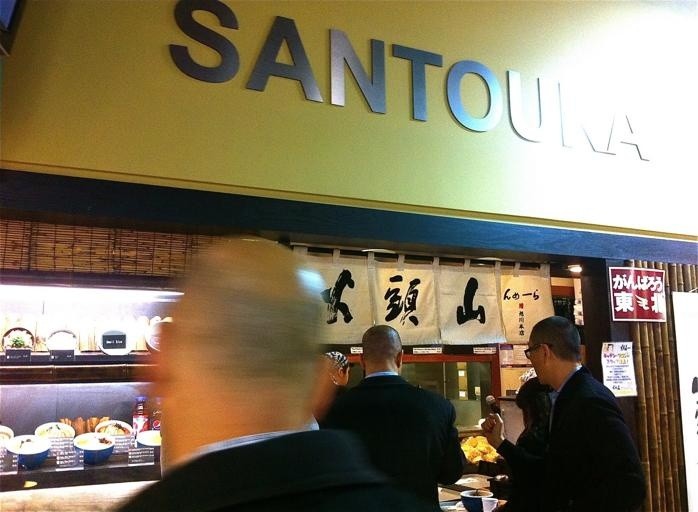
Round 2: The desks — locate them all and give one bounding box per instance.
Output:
[438,473,510,512]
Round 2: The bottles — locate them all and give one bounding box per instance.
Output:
[131,396,151,437]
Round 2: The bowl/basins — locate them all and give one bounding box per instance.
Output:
[6,434,50,467]
[460,490,494,512]
[34,421,75,440]
[73,432,115,465]
[137,429,160,462]
[94,419,132,437]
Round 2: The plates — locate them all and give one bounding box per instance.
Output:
[45,329,78,353]
[98,329,134,356]
[1,326,35,353]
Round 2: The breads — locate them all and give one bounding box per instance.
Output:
[461,435,500,465]
[60,416,111,438]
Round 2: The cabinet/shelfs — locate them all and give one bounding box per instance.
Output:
[0,346,163,489]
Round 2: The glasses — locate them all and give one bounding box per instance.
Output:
[524,343,551,359]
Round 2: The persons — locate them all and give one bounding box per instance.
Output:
[324,323,467,511]
[478,378,561,512]
[107,233,426,511]
[522,315,648,510]
[308,347,357,434]
